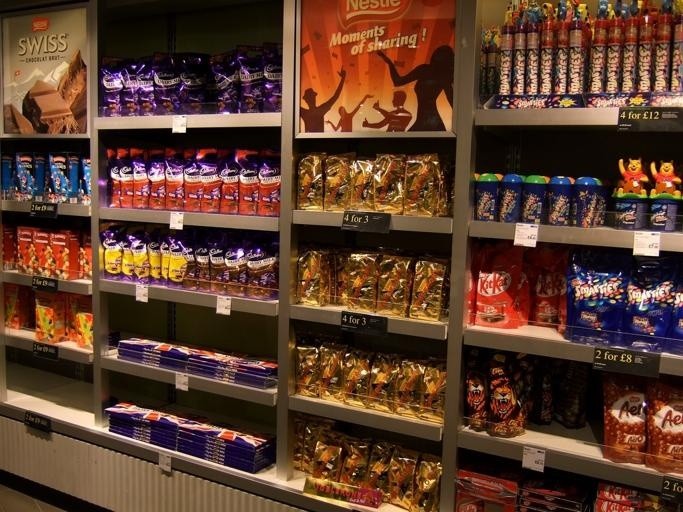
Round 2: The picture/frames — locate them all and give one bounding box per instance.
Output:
[294,0,455,138]
[0,2,90,138]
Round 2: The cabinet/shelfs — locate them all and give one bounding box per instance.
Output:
[0,0,683,509]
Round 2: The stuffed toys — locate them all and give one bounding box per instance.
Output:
[616,158,647,195]
[650,159,681,196]
